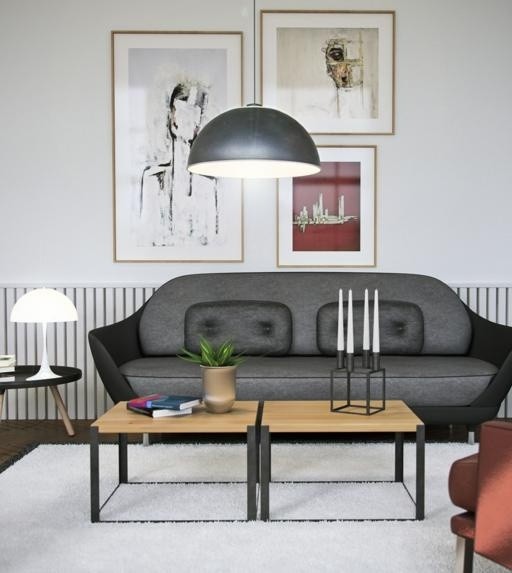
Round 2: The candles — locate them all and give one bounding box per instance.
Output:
[362,287,370,351]
[371,286,381,353]
[346,288,355,354]
[336,288,345,352]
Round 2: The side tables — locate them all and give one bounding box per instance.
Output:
[0,363,83,437]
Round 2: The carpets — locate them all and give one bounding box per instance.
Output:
[1,439,512,572]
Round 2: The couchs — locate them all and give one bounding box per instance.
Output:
[85,269,512,447]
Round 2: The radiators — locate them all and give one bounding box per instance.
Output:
[0,282,511,420]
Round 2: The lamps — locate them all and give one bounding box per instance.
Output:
[7,285,81,381]
[185,1,324,182]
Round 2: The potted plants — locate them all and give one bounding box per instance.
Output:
[173,329,250,414]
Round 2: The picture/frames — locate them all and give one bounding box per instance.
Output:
[108,27,247,268]
[273,143,381,269]
[257,6,398,138]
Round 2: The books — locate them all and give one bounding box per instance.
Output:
[1,375,15,382]
[130,393,202,409]
[0,354,16,366]
[127,402,193,417]
[1,366,16,373]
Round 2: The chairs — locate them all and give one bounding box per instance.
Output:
[446,415,512,571]
